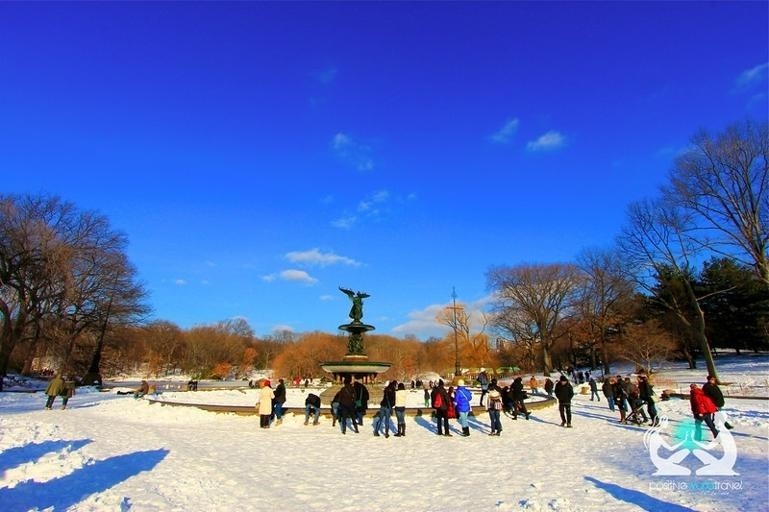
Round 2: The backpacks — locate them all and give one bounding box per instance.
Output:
[433,393,443,408]
[490,397,502,410]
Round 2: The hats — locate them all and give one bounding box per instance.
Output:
[264,381,270,385]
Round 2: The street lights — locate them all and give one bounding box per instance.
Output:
[599,361,605,383]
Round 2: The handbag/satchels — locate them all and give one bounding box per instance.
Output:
[520,391,527,398]
[469,401,472,411]
[355,400,362,408]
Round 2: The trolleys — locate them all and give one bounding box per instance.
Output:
[623,402,648,428]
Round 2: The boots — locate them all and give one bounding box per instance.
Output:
[401,424,405,436]
[394,424,401,437]
[462,427,469,436]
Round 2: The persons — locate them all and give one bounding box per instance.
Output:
[565,365,592,384]
[530,376,538,396]
[589,378,600,400]
[702,376,734,429]
[478,371,530,435]
[40,367,54,376]
[690,384,719,441]
[304,394,321,425]
[331,376,472,436]
[188,380,197,391]
[248,374,308,427]
[602,375,660,427]
[44,374,65,409]
[134,381,162,399]
[555,376,574,426]
[59,377,75,410]
